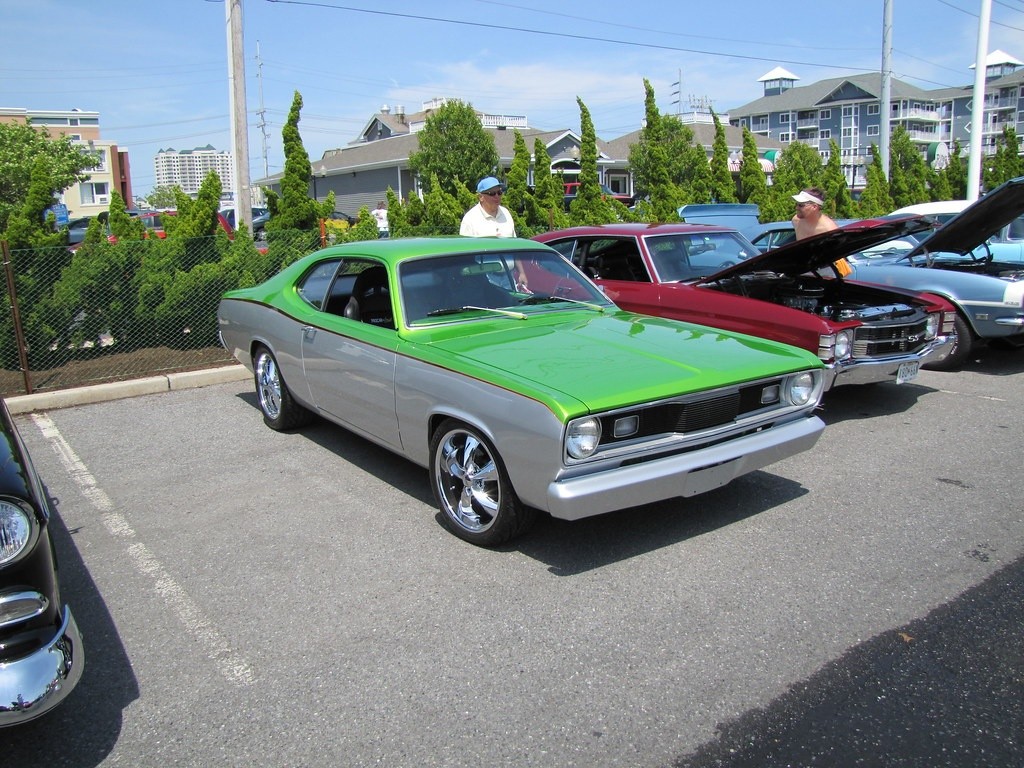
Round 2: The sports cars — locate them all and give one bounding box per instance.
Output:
[668,173,1024,370]
[215,235,828,548]
[508,211,957,393]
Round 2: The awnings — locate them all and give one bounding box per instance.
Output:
[708,158,773,172]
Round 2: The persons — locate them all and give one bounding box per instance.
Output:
[792,189,840,276]
[371,201,390,238]
[459,177,528,292]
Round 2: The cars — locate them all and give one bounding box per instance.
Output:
[883,198,1024,265]
[329,210,355,228]
[60,215,94,246]
[96,201,271,244]
[0,394,86,745]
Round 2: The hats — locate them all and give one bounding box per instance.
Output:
[792,190,823,206]
[477,177,503,193]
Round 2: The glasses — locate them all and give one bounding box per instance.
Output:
[797,203,814,207]
[481,190,502,196]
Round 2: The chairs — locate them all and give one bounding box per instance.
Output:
[344,266,421,330]
[436,262,518,310]
[656,249,691,275]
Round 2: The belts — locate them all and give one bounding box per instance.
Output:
[493,269,515,276]
